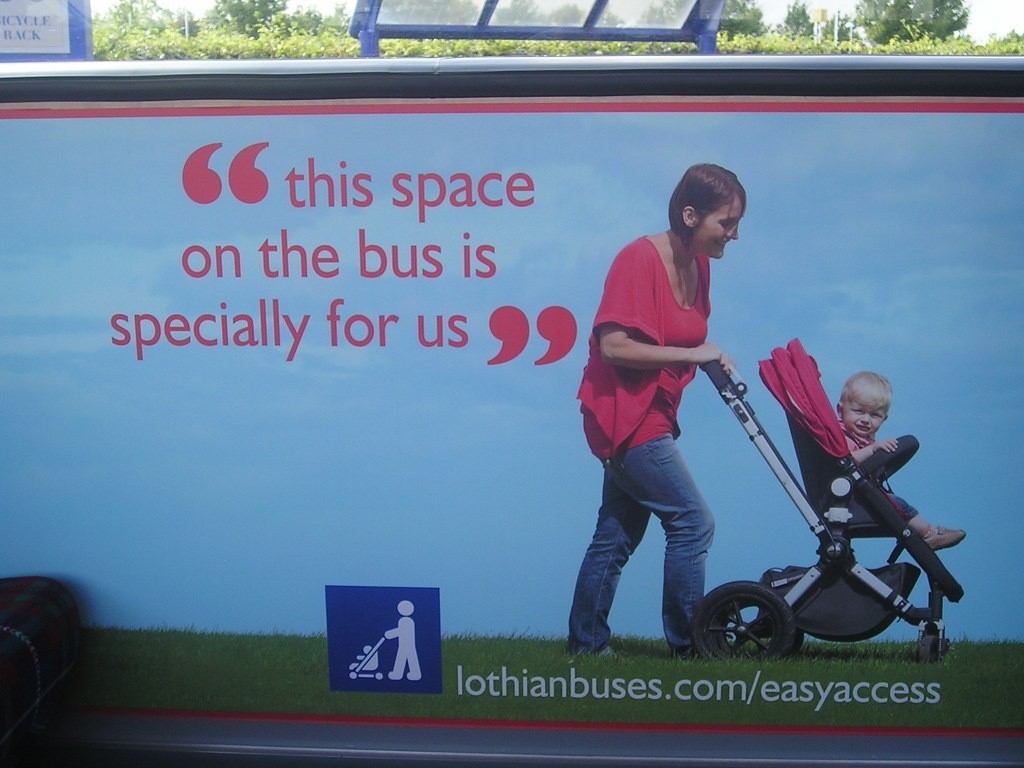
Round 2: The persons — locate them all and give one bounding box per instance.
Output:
[836,371,966,551]
[567,163,747,656]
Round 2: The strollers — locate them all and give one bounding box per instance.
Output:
[690,338,970,664]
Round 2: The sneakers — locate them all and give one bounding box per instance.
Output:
[925,526,966,550]
